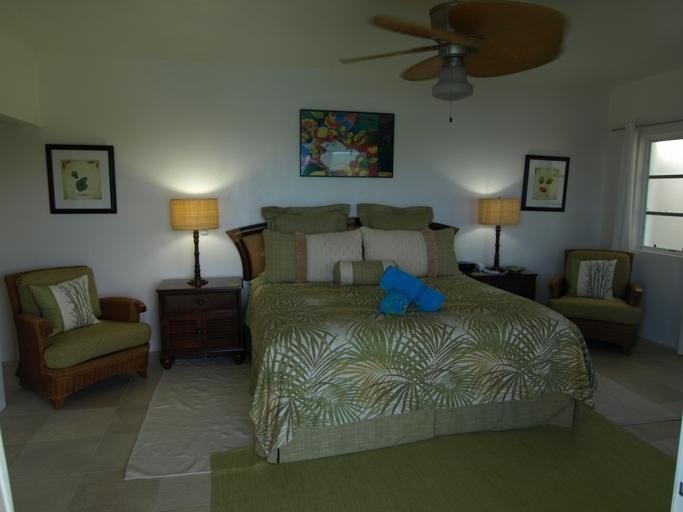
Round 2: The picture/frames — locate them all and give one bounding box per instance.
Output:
[45,143,116,214]
[521,155,571,211]
[300,108,395,177]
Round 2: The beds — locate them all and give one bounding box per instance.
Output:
[226,220,595,464]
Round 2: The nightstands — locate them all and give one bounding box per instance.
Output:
[156,277,245,369]
[469,266,537,301]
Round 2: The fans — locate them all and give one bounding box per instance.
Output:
[340,9,562,82]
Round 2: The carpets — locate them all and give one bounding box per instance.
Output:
[125,356,680,479]
[209,403,676,512]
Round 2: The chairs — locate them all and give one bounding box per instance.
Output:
[4,265,152,409]
[548,248,644,355]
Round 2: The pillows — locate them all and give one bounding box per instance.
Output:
[262,203,459,313]
[577,259,617,300]
[28,274,99,337]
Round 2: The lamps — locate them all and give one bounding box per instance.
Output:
[479,197,521,272]
[431,45,472,122]
[171,198,219,286]
